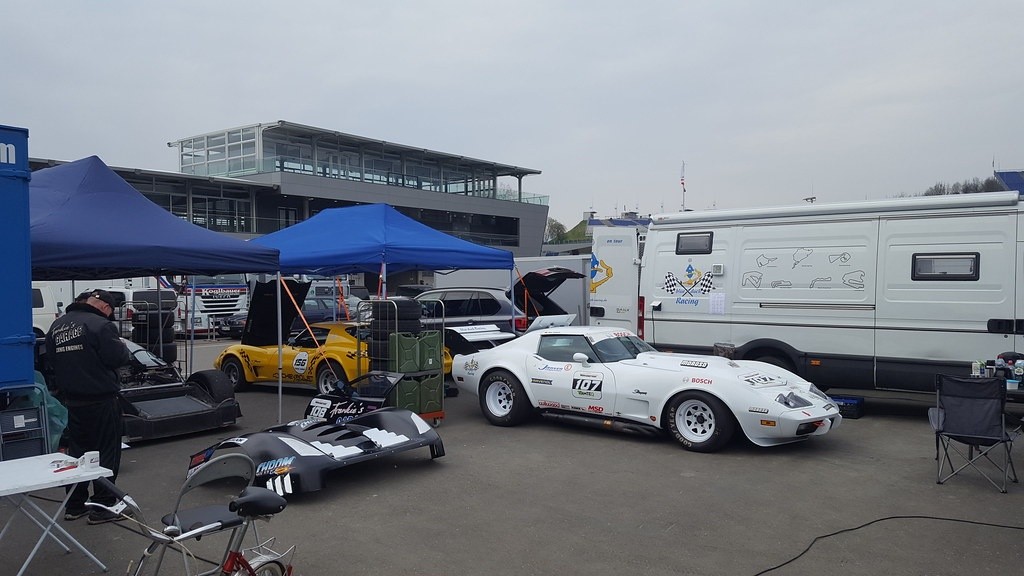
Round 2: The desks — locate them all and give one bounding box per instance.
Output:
[966,389,1024,461]
[0,453,114,576]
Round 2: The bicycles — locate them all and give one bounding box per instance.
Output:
[85,475,294,576]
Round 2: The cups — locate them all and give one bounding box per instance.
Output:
[1006,379,1020,390]
[85,450,99,472]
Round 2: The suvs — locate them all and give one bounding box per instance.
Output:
[358,266,588,352]
[217,296,357,338]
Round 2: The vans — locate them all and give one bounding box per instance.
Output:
[31,285,62,339]
[65,287,182,336]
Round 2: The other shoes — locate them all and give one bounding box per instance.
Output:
[87,506,133,524]
[65,505,90,518]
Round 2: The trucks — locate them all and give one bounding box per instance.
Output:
[131,272,250,338]
[253,272,352,316]
[591,190,1023,394]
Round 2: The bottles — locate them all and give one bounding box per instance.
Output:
[996,356,1004,366]
[1007,361,1015,371]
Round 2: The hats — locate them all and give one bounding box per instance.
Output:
[90,289,116,321]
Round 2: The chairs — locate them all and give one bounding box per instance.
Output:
[155,452,262,576]
[0,384,51,461]
[928,368,1021,494]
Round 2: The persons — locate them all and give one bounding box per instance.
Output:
[46,288,133,525]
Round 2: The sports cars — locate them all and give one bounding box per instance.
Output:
[446,324,842,450]
[213,318,454,396]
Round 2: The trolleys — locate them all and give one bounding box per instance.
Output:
[354,296,446,426]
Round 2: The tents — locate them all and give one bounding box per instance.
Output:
[28,155,282,426]
[189,204,515,374]
